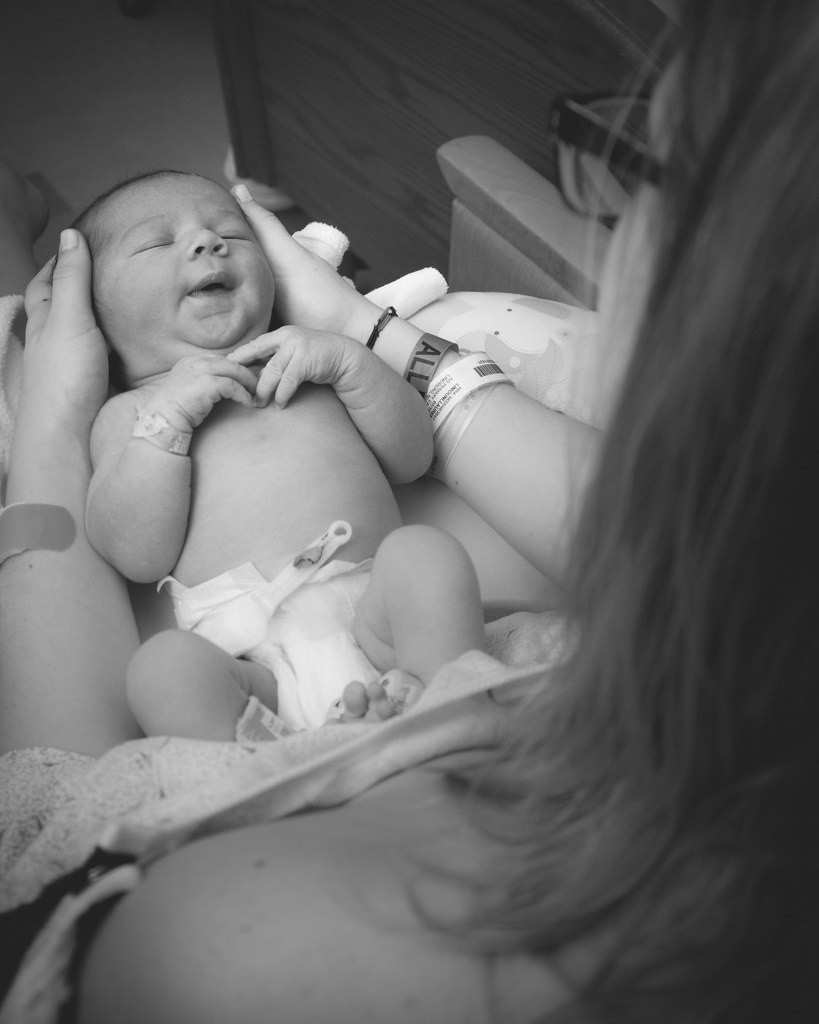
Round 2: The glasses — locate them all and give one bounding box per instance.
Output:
[548,87,681,221]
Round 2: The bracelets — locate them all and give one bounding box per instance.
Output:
[131,409,192,457]
[0,501,76,568]
[402,332,459,401]
[425,350,514,482]
[366,306,399,349]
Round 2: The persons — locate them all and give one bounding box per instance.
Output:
[49,166,487,745]
[0,0,819,1024]
